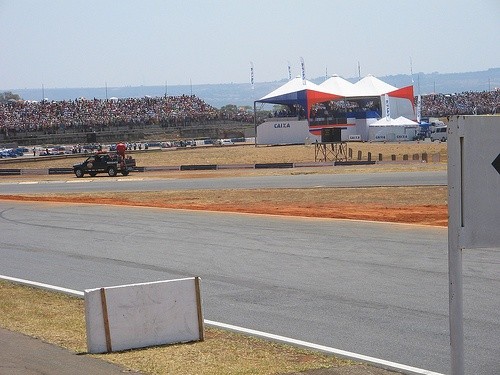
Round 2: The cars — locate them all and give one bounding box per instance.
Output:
[1,137,232,160]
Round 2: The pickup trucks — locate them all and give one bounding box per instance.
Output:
[73,152,136,177]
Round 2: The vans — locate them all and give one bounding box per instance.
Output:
[430,125,447,143]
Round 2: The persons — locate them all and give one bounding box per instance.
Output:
[0,89,500,168]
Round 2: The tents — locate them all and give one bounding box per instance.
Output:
[253,74,421,148]
